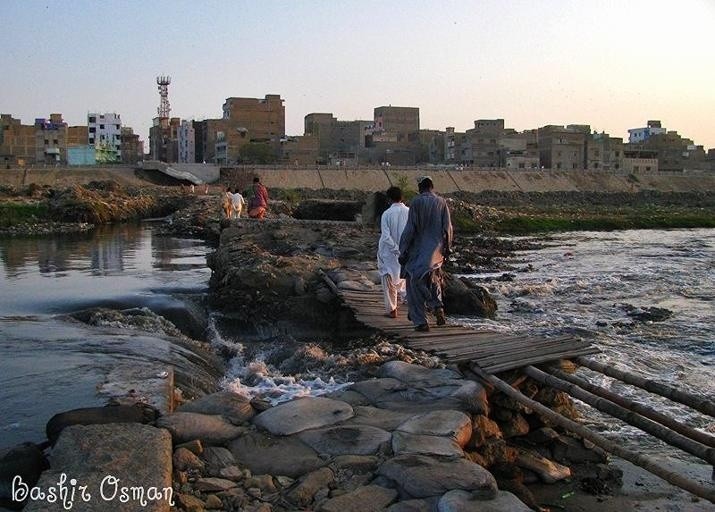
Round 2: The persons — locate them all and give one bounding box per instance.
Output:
[376,187,410,318]
[247,177,268,219]
[398,175,454,332]
[223,187,245,219]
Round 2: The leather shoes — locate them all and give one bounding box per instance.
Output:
[436,308,445,325]
[416,325,429,331]
[389,309,397,317]
